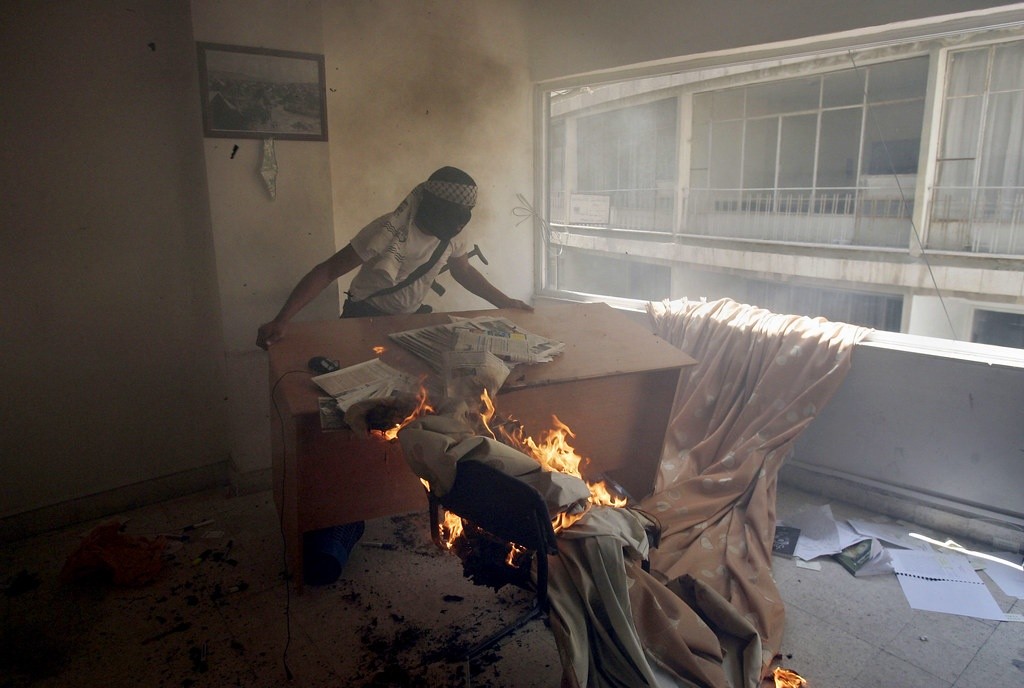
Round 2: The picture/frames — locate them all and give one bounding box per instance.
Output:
[197,40,329,142]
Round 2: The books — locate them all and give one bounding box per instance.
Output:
[772,526,801,559]
[887,548,1009,621]
[832,537,894,578]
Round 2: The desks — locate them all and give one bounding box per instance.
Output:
[267,302,697,591]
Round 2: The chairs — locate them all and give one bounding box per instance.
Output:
[432,462,660,666]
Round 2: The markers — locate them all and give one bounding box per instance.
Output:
[157,518,214,541]
[364,540,392,548]
[193,540,248,595]
